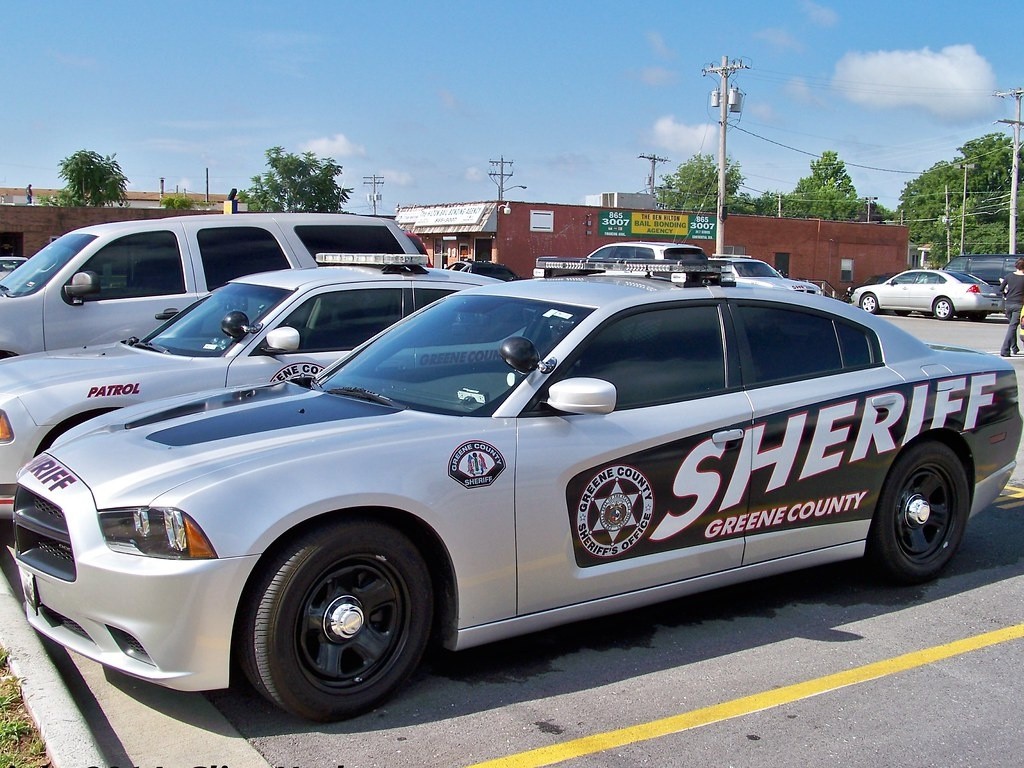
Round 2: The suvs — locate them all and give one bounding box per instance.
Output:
[576,240,710,277]
[941,254,1023,291]
[0,210,435,376]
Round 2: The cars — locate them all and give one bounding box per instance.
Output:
[850,268,1009,321]
[1,252,509,540]
[709,256,824,297]
[12,255,1022,726]
[444,260,521,283]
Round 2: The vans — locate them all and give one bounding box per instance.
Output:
[403,232,436,270]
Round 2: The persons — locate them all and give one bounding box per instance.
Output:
[26,185,32,204]
[997,257,1024,357]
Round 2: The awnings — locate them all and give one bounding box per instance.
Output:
[393,202,497,234]
